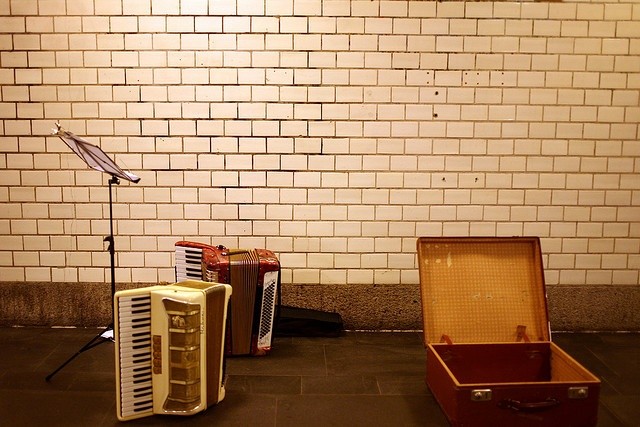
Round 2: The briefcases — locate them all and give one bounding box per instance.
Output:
[416,236,602,427]
